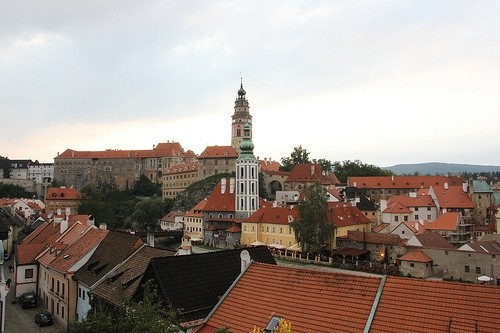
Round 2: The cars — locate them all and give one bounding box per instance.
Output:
[34,311,53,327]
[19,291,38,309]
